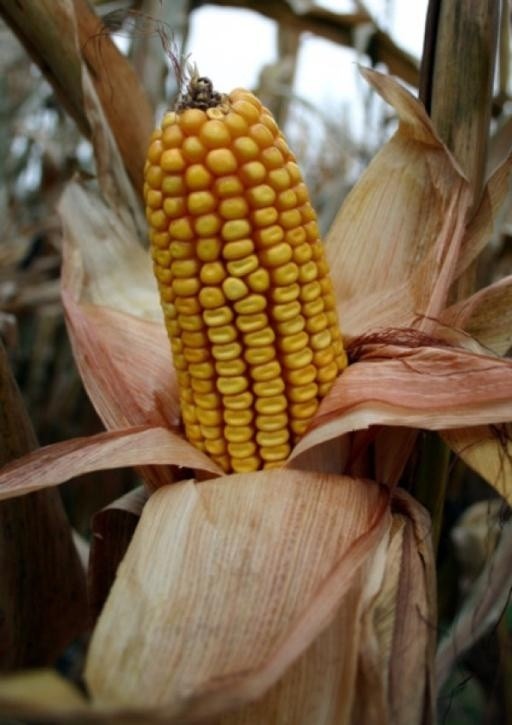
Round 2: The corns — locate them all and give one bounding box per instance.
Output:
[143,70,351,476]
